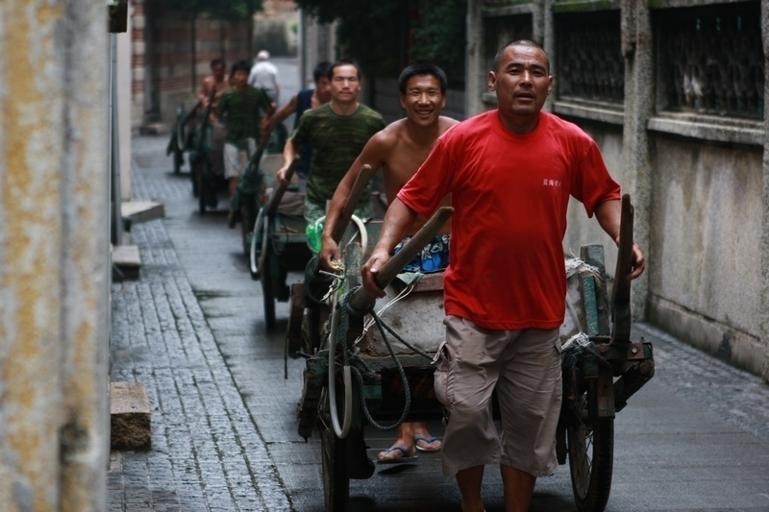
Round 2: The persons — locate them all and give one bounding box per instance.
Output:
[359,37,651,511]
[257,60,336,195]
[210,57,277,223]
[194,57,231,129]
[274,58,387,259]
[246,49,282,119]
[317,59,463,462]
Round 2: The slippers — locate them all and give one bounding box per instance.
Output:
[414,434,442,451]
[376,442,418,464]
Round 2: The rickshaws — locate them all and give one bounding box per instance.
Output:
[288,195,654,511]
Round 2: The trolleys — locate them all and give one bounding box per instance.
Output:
[165,84,287,230]
[247,152,325,333]
